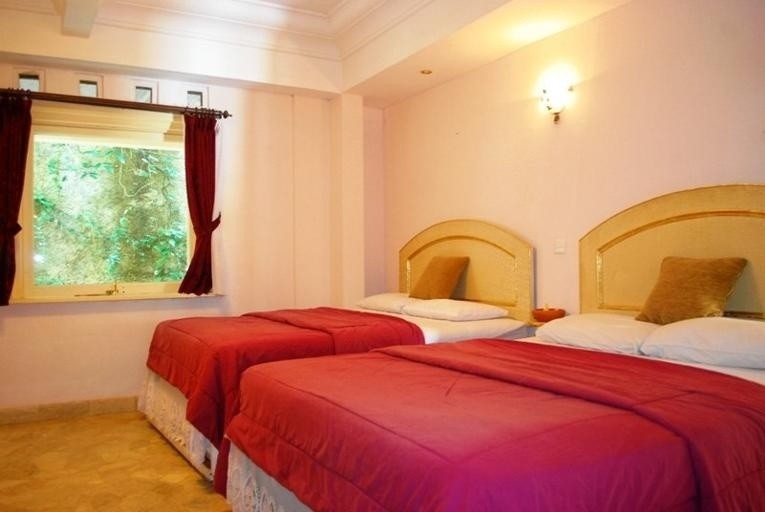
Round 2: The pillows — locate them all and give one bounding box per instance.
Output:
[402,298,508,322]
[638,317,765,373]
[355,292,425,316]
[634,257,749,328]
[535,314,661,357]
[409,257,470,300]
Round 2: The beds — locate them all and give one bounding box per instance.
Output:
[226,184,764,509]
[147,220,537,484]
